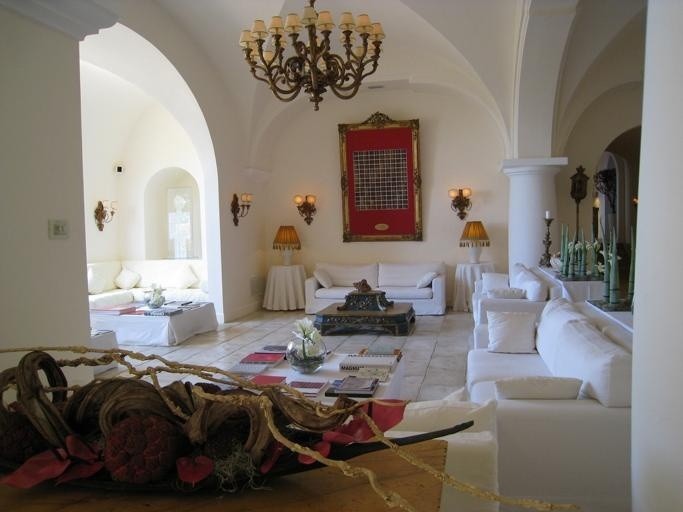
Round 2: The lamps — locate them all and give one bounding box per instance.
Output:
[458,220,489,261]
[229,191,257,227]
[270,224,301,263]
[447,188,473,220]
[238,0,385,110]
[569,165,589,243]
[94,197,118,231]
[293,192,318,226]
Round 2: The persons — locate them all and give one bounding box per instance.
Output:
[167,194,191,261]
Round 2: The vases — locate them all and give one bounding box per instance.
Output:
[285,338,325,374]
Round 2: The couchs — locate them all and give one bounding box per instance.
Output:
[469,263,558,346]
[457,298,630,511]
[303,262,447,316]
[86,260,208,306]
[383,402,499,510]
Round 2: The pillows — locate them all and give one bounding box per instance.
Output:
[484,287,524,298]
[310,267,332,289]
[485,306,535,352]
[168,265,196,291]
[481,271,509,287]
[495,374,581,401]
[418,270,438,287]
[114,267,139,291]
[87,269,113,292]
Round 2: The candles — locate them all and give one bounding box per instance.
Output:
[544,210,551,219]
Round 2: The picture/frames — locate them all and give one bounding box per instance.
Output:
[337,109,421,243]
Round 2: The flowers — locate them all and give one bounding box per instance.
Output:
[293,319,319,340]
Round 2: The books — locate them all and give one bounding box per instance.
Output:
[212,342,403,400]
[91,298,207,317]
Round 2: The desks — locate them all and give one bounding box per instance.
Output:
[259,262,306,314]
[451,262,494,311]
[525,258,605,306]
[1,434,452,510]
[90,300,216,347]
[574,296,633,351]
